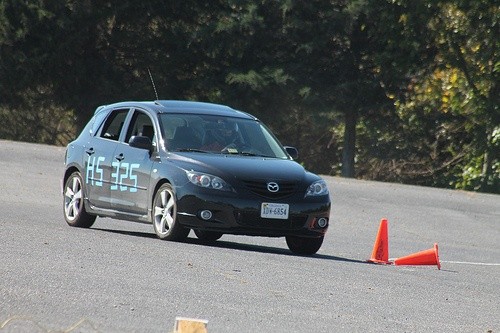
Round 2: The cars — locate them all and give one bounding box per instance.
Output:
[61,99,331,256]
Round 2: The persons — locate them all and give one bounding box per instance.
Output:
[200,121,249,154]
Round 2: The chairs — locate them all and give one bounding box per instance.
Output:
[116,121,138,142]
[174,127,202,150]
[142,125,165,141]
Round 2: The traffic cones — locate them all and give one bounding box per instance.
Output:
[367,219,393,265]
[393,243,441,271]
